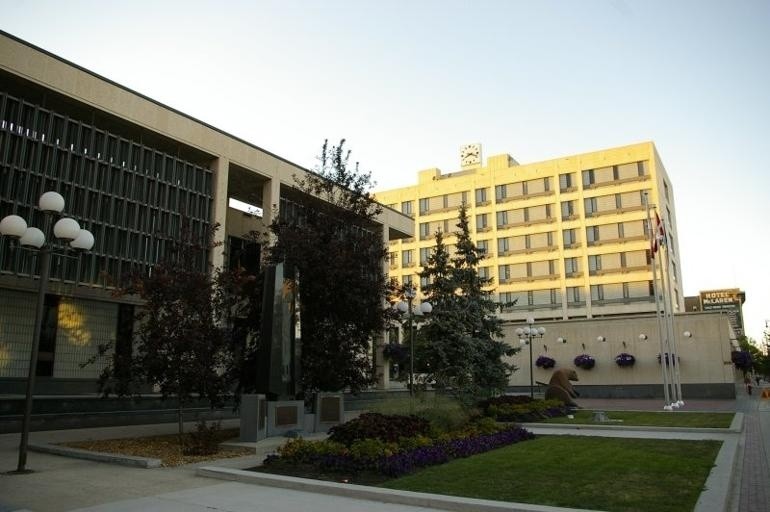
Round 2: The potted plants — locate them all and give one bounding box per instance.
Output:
[348,438,395,483]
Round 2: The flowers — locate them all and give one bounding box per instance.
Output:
[573,353,595,370]
[656,352,680,365]
[535,356,555,370]
[615,352,634,368]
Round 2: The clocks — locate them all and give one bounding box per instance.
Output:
[460,143,481,167]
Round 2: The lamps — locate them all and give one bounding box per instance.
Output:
[639,334,648,340]
[519,338,529,344]
[597,335,606,342]
[557,337,567,343]
[683,331,692,337]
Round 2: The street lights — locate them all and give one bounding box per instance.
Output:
[393,284,433,399]
[0,187,100,481]
[515,314,547,401]
